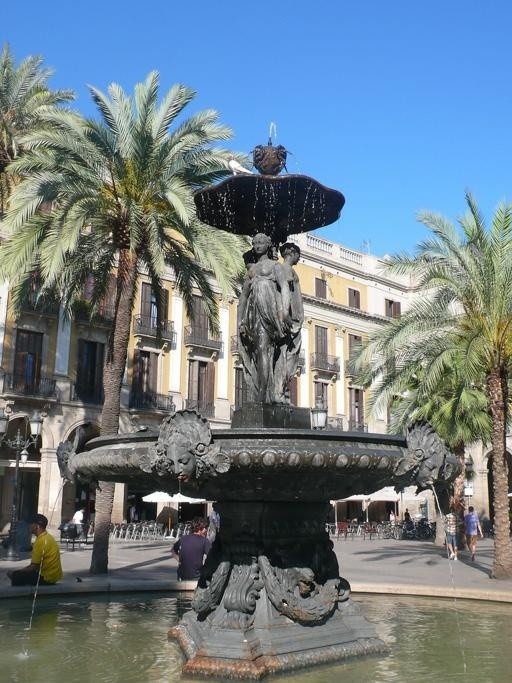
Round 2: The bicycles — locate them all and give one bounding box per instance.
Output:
[375,519,436,540]
[108,520,192,542]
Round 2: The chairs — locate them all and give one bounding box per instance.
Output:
[363,521,380,539]
[336,521,356,540]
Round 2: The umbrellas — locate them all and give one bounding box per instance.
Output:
[142,492,206,536]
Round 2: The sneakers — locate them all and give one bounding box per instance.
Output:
[447,551,477,562]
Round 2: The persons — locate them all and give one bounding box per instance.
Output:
[463,506,484,560]
[5,514,64,585]
[58,505,95,540]
[170,502,220,581]
[390,510,395,521]
[442,506,460,561]
[124,501,156,523]
[136,408,232,484]
[404,508,412,520]
[392,423,453,489]
[235,232,304,408]
[54,440,76,484]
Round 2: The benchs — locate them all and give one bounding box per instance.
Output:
[60,523,94,552]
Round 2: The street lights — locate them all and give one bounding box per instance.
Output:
[0,409,44,561]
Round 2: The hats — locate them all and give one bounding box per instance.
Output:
[25,513,49,525]
